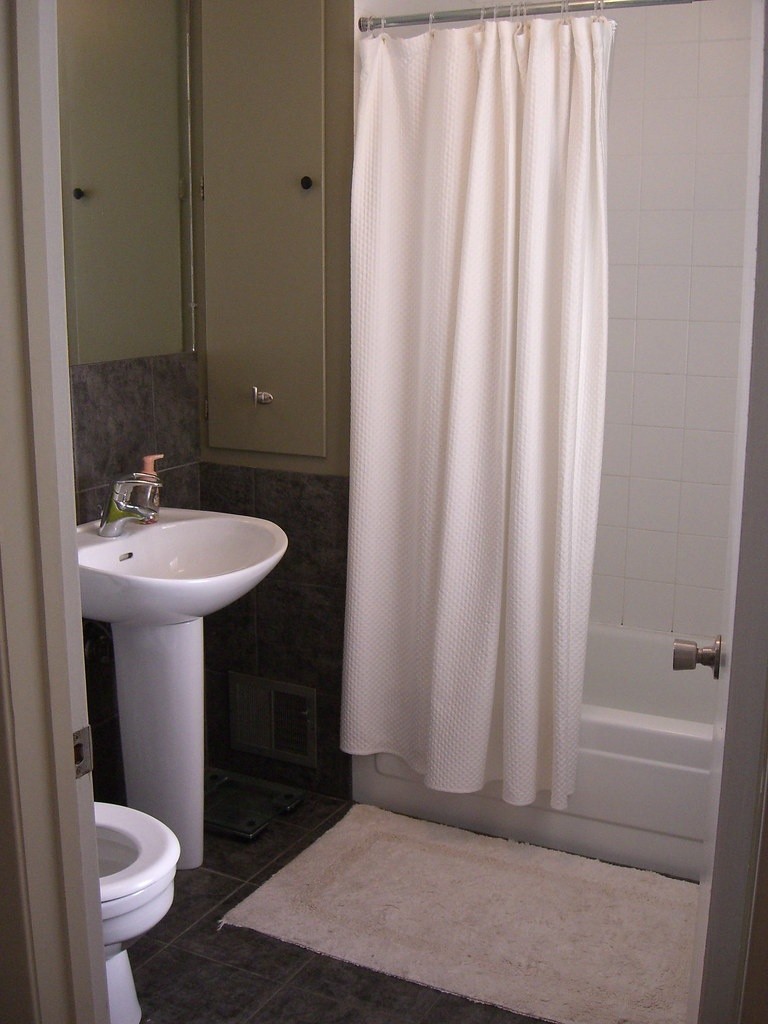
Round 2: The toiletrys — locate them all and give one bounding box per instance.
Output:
[132,454,164,514]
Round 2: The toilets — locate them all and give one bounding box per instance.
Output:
[93,800,182,1024]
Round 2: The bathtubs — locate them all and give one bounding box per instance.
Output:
[353,620,722,880]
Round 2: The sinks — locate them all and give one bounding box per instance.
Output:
[76,507,289,624]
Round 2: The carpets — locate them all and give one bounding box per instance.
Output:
[220,803,703,1024]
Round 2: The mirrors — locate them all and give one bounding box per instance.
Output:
[56,0,186,365]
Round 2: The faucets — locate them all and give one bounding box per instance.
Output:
[97,472,166,538]
